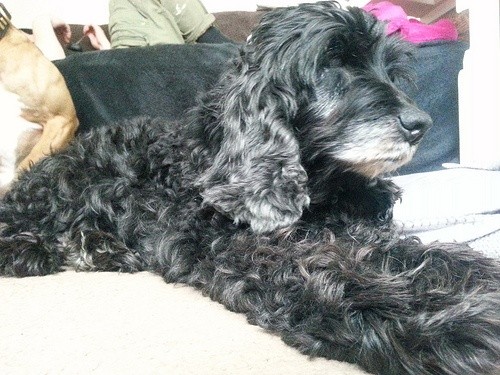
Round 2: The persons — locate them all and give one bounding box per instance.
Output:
[0,1,79,202]
[50,22,112,54]
[108,0,236,46]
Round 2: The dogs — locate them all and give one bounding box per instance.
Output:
[2,0,500,375]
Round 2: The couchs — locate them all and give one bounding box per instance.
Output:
[44,1,469,177]
